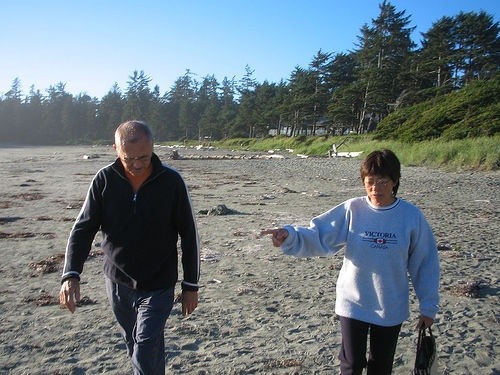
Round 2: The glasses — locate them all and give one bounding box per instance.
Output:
[366,179,391,186]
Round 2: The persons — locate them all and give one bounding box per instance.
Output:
[58,120,201,375]
[259,148,440,375]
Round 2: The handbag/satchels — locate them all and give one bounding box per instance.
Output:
[413,319,438,375]
